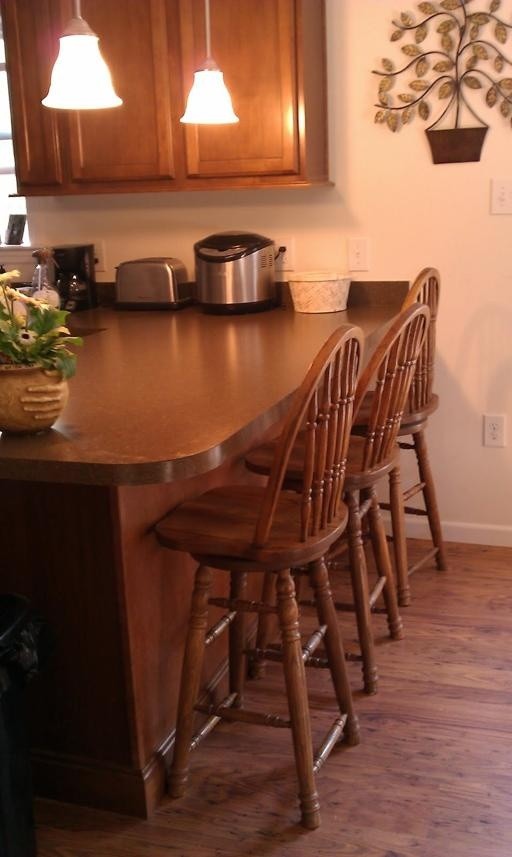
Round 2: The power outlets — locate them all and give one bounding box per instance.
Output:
[270,236,294,271]
[481,411,505,450]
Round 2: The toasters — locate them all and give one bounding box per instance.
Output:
[113,256,191,312]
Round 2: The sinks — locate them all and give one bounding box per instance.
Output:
[61,326,107,339]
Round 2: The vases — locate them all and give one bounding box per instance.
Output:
[0,353,72,438]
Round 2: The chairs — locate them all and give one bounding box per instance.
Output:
[318,262,449,608]
[152,321,365,837]
[240,297,434,695]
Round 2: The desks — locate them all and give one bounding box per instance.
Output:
[2,279,416,818]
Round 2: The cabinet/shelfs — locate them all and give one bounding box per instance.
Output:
[1,0,185,197]
[173,0,334,193]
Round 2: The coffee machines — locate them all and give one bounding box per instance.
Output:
[53,240,101,312]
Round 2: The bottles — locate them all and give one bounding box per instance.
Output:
[28,264,61,314]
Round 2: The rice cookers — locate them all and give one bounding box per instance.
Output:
[194,230,277,312]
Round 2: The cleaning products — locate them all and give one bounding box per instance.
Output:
[28,248,56,293]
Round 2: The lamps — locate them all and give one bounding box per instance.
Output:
[36,0,124,111]
[178,0,240,125]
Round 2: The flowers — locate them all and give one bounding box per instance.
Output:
[0,255,87,386]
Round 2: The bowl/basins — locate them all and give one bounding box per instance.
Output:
[287,271,351,313]
